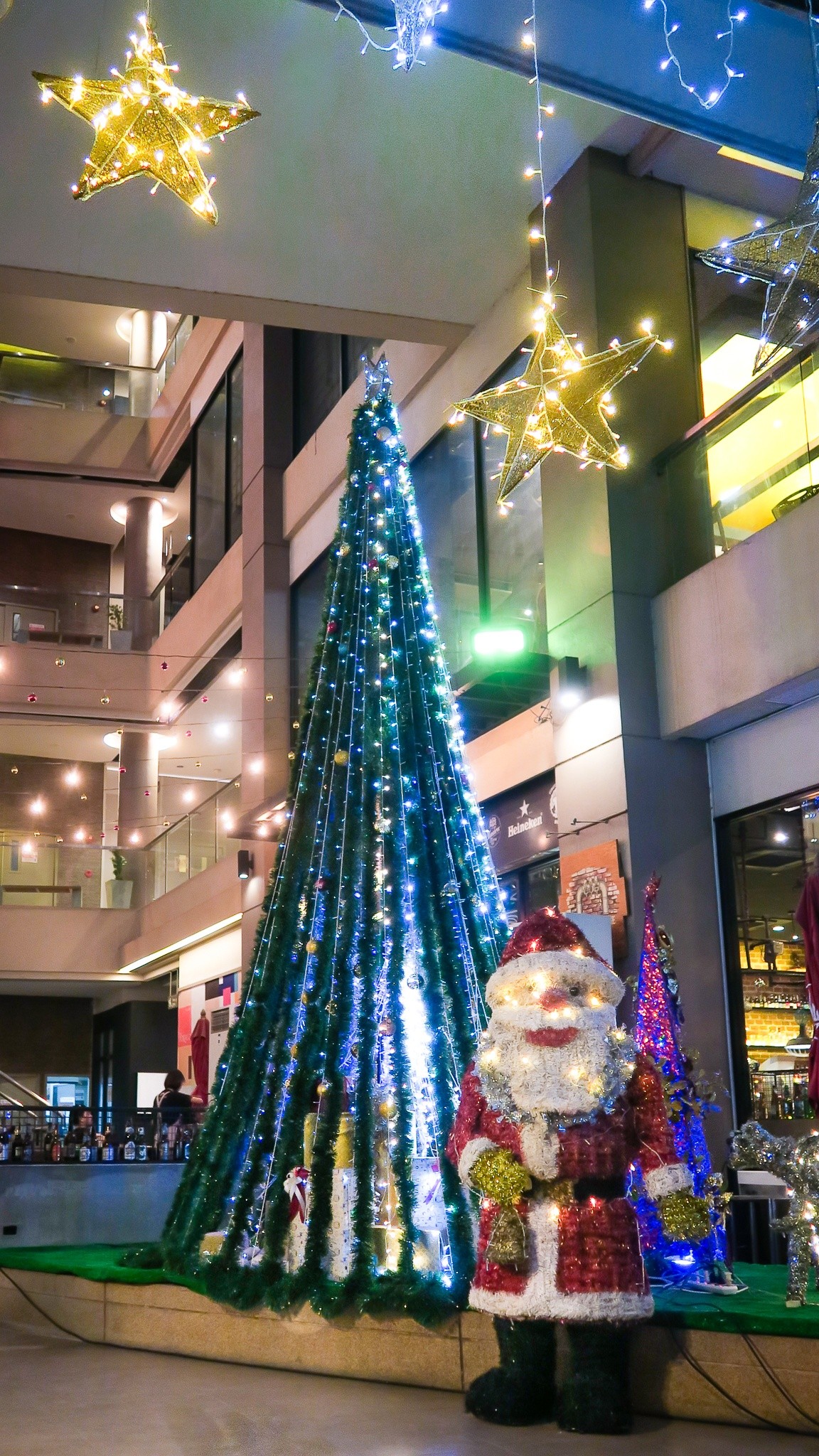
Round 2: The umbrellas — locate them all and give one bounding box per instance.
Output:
[792,854,819,1117]
[190,1010,209,1108]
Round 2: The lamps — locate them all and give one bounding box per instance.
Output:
[784,1009,812,1058]
[238,850,254,879]
[558,656,588,707]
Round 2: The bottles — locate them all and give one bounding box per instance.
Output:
[123,1117,147,1162]
[782,1083,814,1120]
[43,1123,91,1163]
[771,1091,779,1118]
[758,1092,765,1119]
[0,1126,31,1164]
[102,1123,114,1163]
[174,1126,191,1162]
[157,1123,170,1163]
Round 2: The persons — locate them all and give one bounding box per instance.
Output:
[153,1069,203,1148]
[444,907,709,1434]
[64,1105,105,1147]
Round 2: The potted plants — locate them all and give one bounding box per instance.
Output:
[106,605,133,650]
[106,849,134,909]
